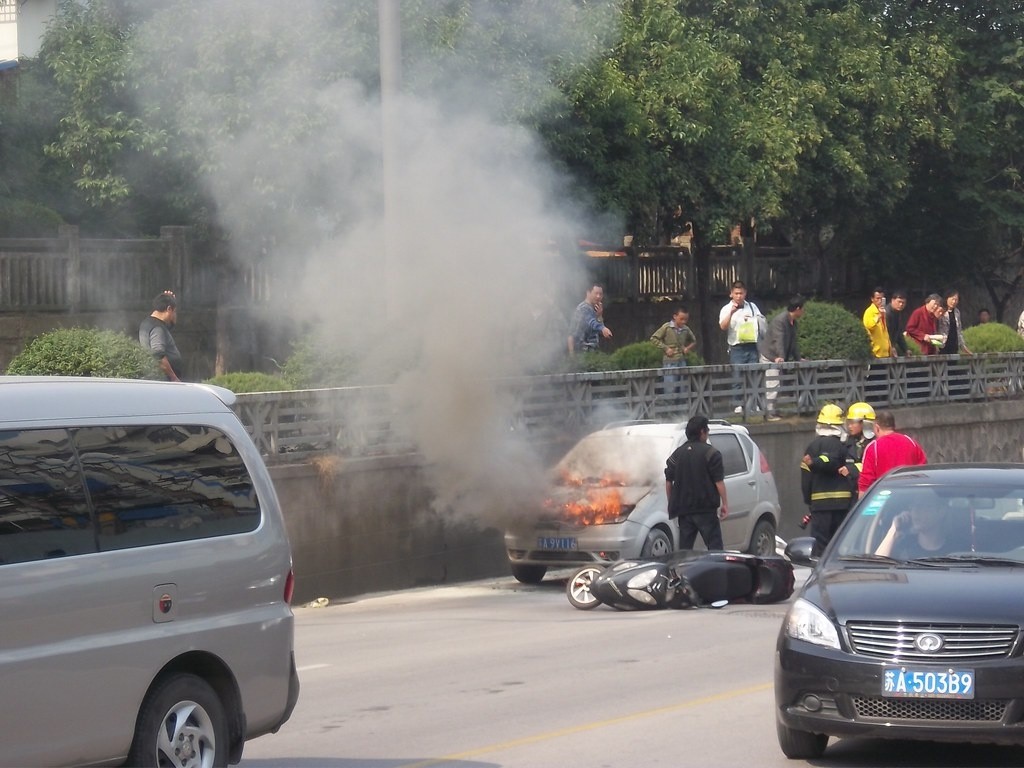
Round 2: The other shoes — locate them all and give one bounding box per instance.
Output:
[766,415,781,422]
[735,406,742,413]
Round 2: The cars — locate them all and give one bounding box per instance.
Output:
[773,459,1024,761]
[503,417,783,585]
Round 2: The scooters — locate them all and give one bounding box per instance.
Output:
[566,545,796,612]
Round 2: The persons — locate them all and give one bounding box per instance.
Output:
[800,404,856,557]
[719,282,768,413]
[532,284,612,414]
[139,292,187,381]
[1017,312,1024,336]
[863,289,913,402]
[905,292,973,364]
[760,298,805,421]
[979,310,991,323]
[804,402,877,505]
[665,415,729,549]
[858,412,928,499]
[650,308,697,404]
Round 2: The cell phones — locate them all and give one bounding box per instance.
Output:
[736,305,744,309]
[882,298,886,309]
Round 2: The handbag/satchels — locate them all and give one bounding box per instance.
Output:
[738,316,758,343]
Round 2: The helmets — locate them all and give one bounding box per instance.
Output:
[847,402,878,420]
[817,405,847,425]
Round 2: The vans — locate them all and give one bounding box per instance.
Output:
[0,375,301,768]
